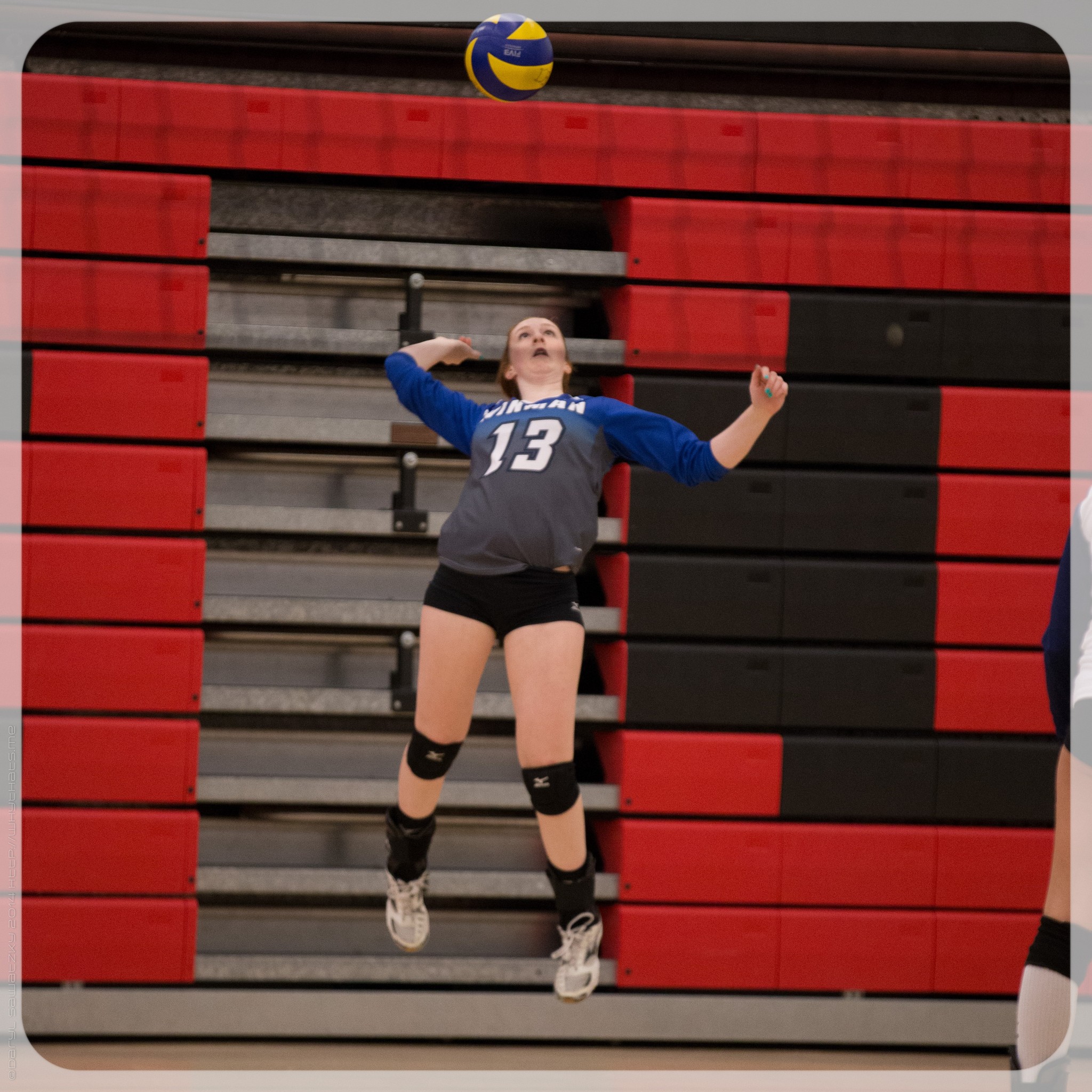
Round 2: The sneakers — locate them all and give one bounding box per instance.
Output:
[555,914,602,1002]
[385,868,430,952]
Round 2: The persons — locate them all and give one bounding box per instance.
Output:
[1011,488,1092,1092]
[383,316,788,1003]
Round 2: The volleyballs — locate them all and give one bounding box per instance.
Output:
[465,13,554,104]
